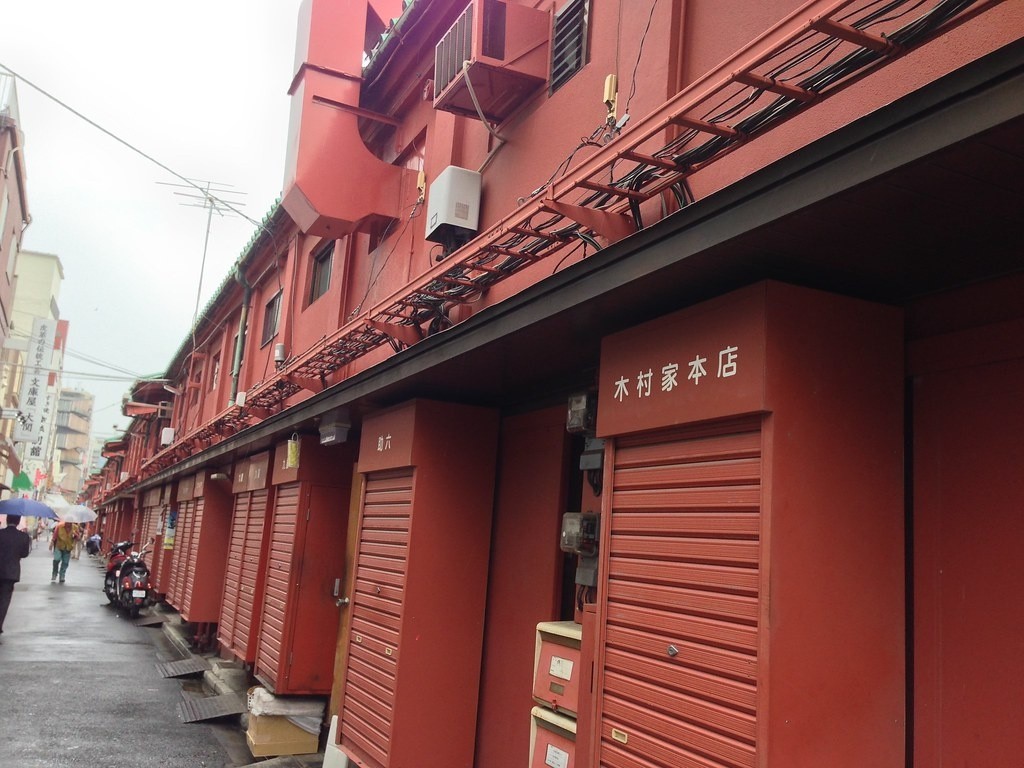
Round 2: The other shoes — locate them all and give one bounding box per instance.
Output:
[51,579,56,583]
[70,555,79,560]
[59,579,65,583]
[89,554,95,558]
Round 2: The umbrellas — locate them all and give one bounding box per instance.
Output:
[48,504,99,522]
[0,498,59,518]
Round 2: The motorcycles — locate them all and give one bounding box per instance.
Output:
[103,531,157,620]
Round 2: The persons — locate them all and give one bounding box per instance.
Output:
[0,513,29,632]
[36,517,87,560]
[49,522,81,582]
